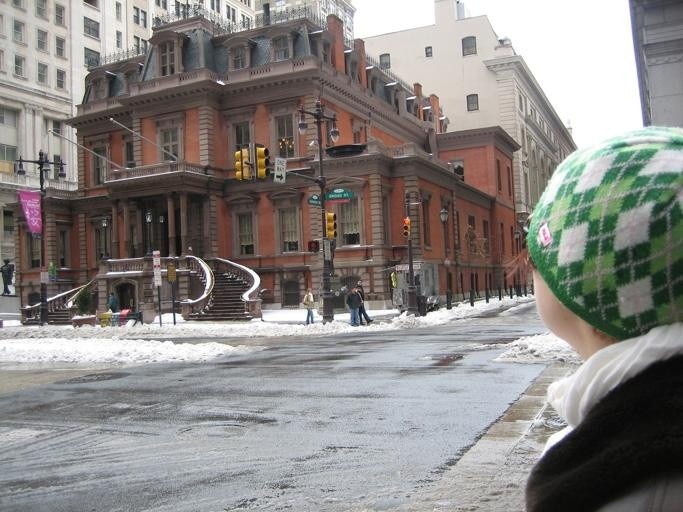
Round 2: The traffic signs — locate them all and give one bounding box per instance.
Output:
[272,158,286,184]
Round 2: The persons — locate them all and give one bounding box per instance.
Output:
[106,293,118,312]
[346,280,374,326]
[1,258,14,295]
[302,288,316,324]
[524,125,682,512]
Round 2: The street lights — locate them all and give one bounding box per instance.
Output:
[144,207,154,255]
[102,217,110,259]
[14,149,67,325]
[513,229,520,296]
[296,100,342,325]
[438,208,451,310]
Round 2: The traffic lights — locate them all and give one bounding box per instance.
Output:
[233,144,252,182]
[324,212,337,239]
[402,218,410,237]
[252,143,269,179]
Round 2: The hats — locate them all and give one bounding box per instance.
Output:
[525,124,683,341]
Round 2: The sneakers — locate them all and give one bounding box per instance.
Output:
[351,319,374,326]
[306,321,315,324]
